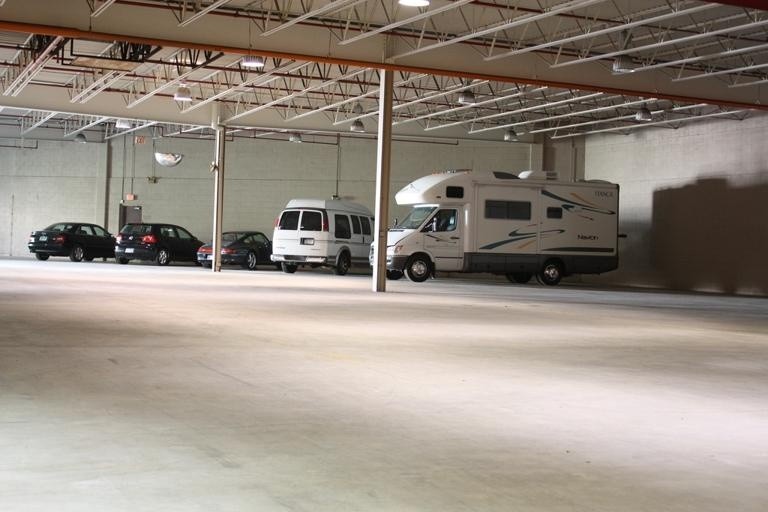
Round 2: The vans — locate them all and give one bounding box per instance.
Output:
[270,199,374,275]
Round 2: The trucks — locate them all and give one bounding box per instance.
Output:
[369,169,620,285]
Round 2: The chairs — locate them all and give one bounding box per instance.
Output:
[446,216,456,231]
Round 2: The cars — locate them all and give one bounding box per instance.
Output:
[28,223,282,270]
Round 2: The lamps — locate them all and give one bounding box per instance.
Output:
[116,120,133,129]
[613,53,636,73]
[635,101,653,121]
[172,76,192,102]
[76,132,87,143]
[350,119,365,133]
[240,10,265,67]
[289,132,301,143]
[398,1,430,7]
[504,126,520,142]
[353,101,365,114]
[458,81,477,104]
